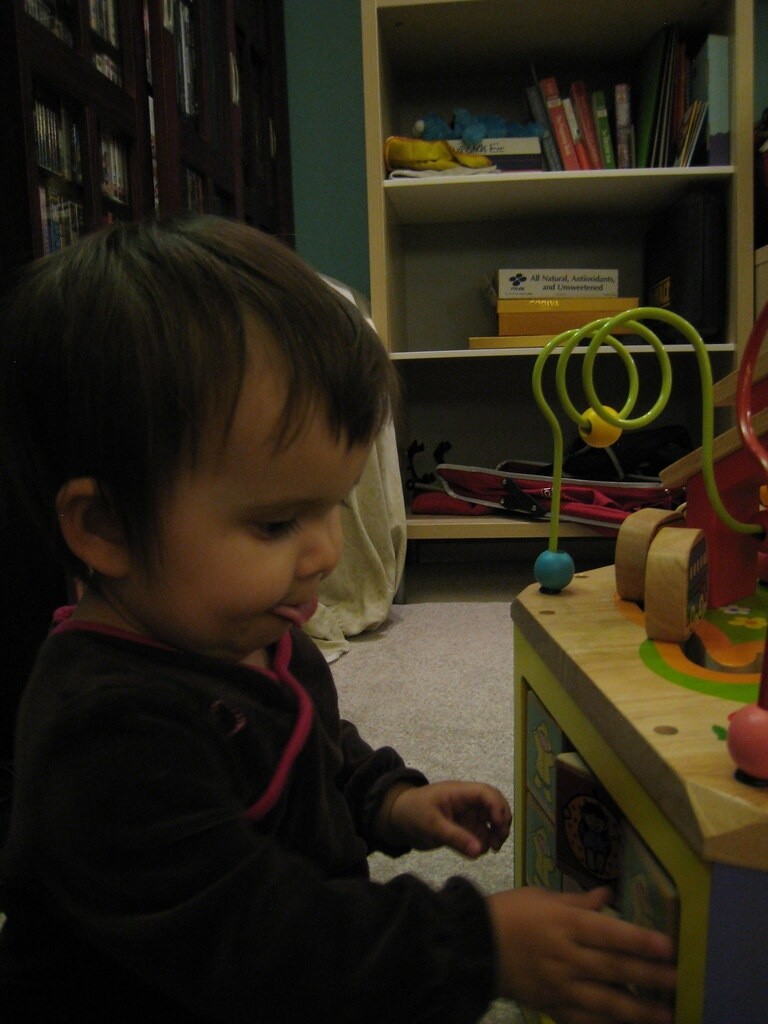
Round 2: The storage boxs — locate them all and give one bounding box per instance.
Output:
[499,269,618,298]
[497,297,639,336]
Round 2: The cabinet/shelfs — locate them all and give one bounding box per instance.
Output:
[363,0,755,602]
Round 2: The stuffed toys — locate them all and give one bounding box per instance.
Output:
[412,108,544,147]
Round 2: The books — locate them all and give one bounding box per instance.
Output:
[20,0,203,257]
[524,26,709,173]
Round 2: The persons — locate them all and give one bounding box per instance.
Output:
[0,214,674,1024]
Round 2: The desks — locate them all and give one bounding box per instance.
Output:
[512,563,768,1024]
